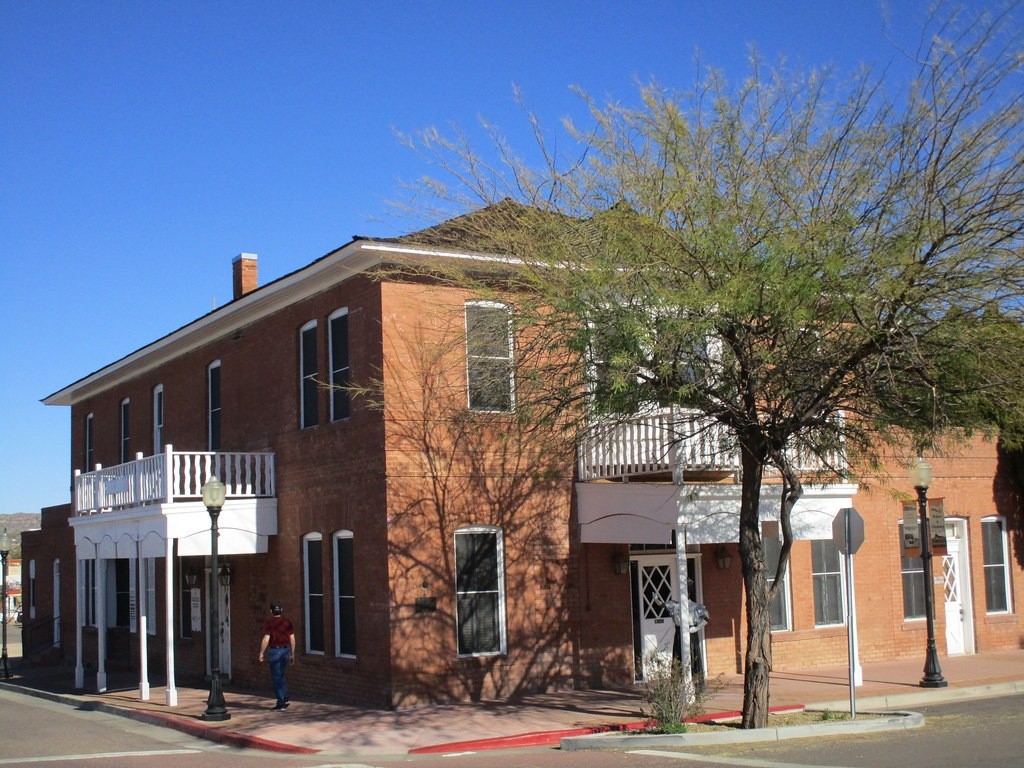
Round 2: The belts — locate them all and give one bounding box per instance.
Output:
[270,645,287,648]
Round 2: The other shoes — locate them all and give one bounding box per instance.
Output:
[271,705,287,711]
[276,701,290,708]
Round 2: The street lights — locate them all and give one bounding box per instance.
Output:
[0,528,13,677]
[201,473,232,721]
[911,456,948,688]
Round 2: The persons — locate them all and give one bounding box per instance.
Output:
[258,600,295,712]
[652,577,709,696]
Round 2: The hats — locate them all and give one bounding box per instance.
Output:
[270,601,282,611]
